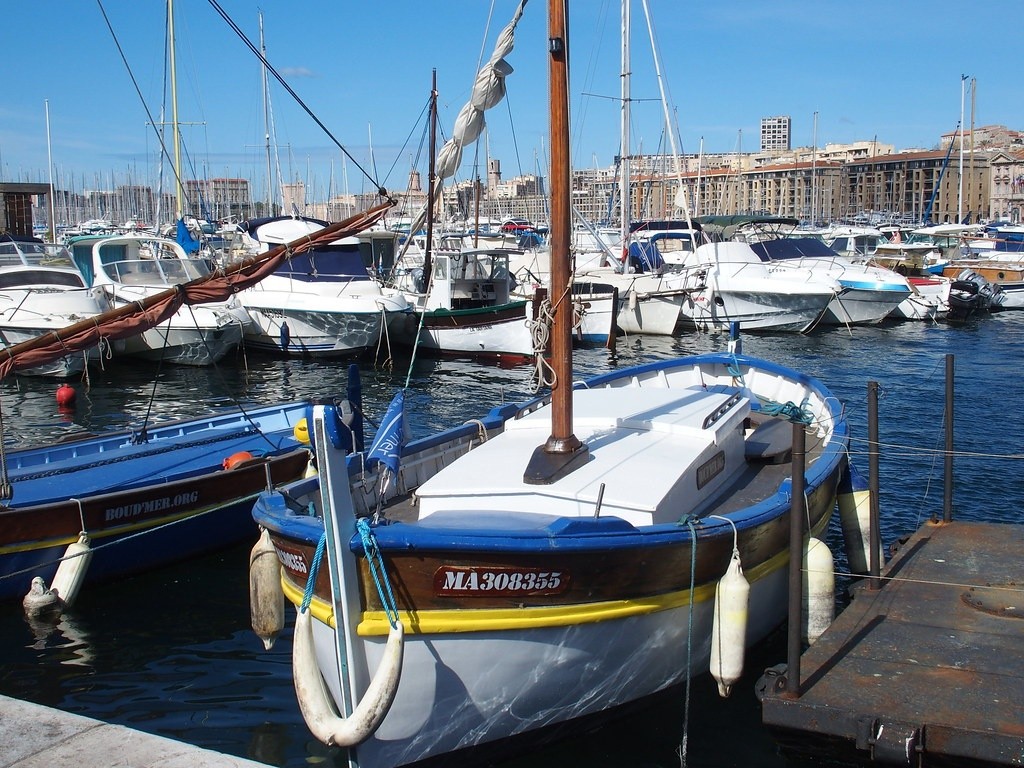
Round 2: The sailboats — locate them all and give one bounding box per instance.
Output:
[249,0,885,768]
[0,0,397,617]
[0,0,1023,406]
